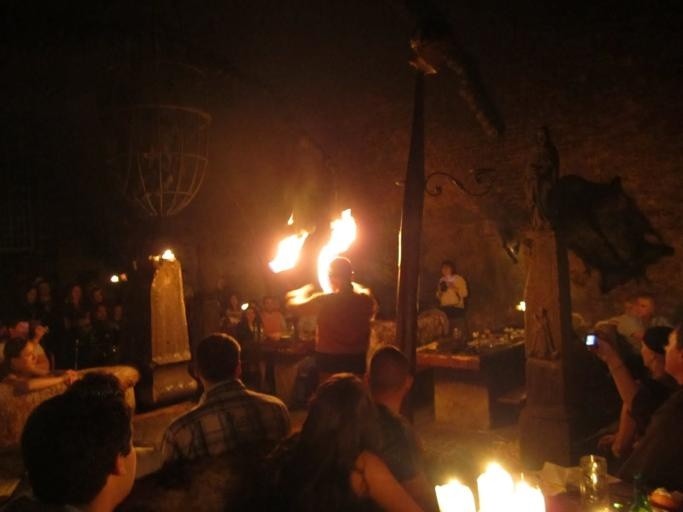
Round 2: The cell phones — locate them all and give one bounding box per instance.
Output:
[584,333,597,347]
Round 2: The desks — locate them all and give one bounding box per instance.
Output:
[416,342,527,432]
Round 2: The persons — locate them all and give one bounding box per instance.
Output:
[253,345,440,511]
[158,333,294,476]
[221,293,313,365]
[285,256,379,390]
[435,261,468,328]
[13,372,138,512]
[0,273,123,395]
[570,296,683,495]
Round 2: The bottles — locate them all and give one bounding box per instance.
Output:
[630,471,653,512]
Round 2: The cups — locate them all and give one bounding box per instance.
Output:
[578,454,609,512]
